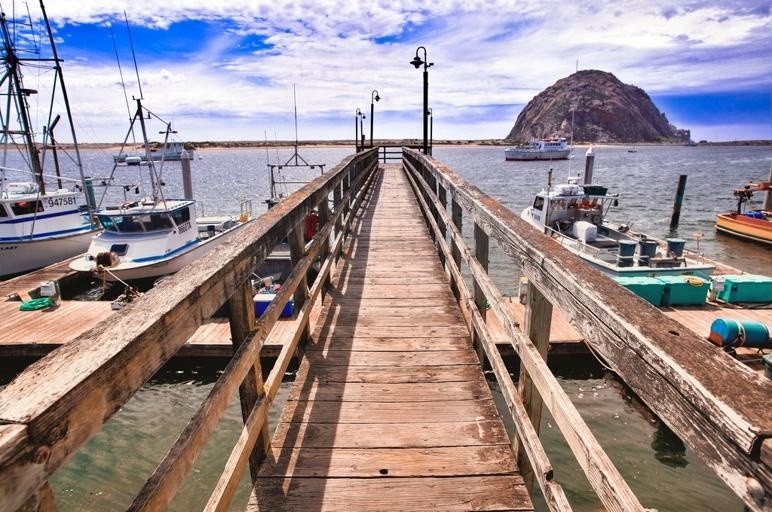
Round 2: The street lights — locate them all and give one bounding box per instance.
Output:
[355,107,361,154]
[427,108,433,157]
[410,45,429,156]
[370,89,381,148]
[360,113,366,152]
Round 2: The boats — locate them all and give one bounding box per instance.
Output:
[522,58,771,384]
[713,168,772,248]
[504,137,570,161]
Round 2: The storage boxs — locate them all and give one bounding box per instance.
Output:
[611,275,772,307]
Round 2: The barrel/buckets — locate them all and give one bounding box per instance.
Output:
[639,238,659,256]
[617,239,638,266]
[666,237,686,256]
